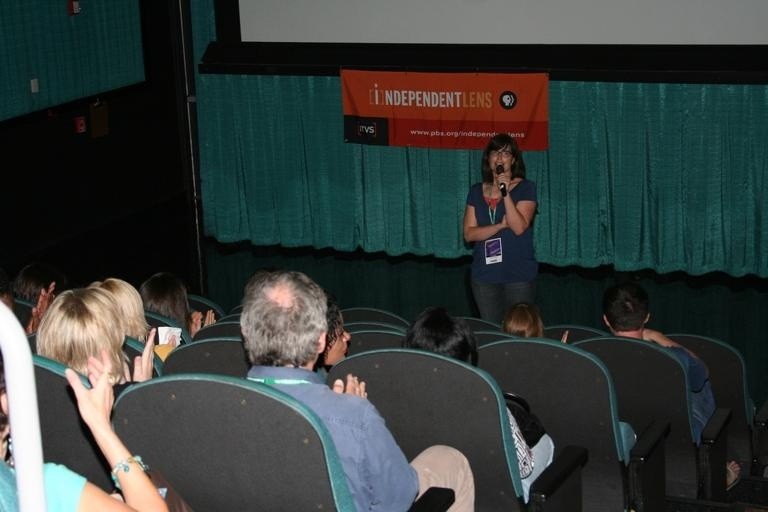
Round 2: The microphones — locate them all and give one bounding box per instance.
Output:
[495,164,507,198]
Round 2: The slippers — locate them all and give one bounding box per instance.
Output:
[720,455,744,495]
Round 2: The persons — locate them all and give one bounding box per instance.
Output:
[503,287,739,491]
[0,261,555,511]
[463,133,540,327]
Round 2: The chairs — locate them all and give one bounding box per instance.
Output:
[576,333,710,510]
[664,333,767,510]
[471,338,627,512]
[3,282,614,486]
[106,373,458,512]
[326,342,587,512]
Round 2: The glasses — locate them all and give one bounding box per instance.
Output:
[489,149,514,158]
